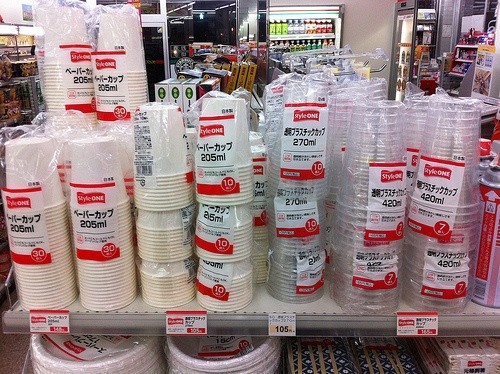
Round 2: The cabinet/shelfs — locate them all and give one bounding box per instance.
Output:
[388,0,439,101]
[268,4,345,83]
[0,263,500,374]
[0,22,46,301]
[441,44,486,97]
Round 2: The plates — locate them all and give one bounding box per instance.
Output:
[31,332,167,374]
[163,335,282,374]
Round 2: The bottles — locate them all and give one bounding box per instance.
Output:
[415,36,419,47]
[270,39,334,52]
[270,20,333,35]
[413,61,418,76]
[397,51,410,90]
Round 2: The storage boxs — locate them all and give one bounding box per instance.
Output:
[154,61,258,128]
[192,42,213,50]
[480,103,500,140]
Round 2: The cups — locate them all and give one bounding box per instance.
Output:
[37,6,147,124]
[4,76,482,314]
